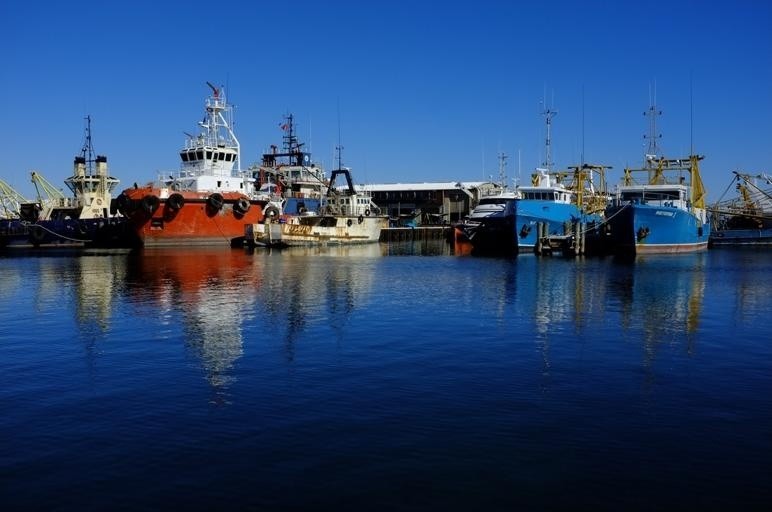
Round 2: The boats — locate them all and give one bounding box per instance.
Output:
[0,73,391,250]
[604,81,711,257]
[450,80,613,257]
[706,169,771,250]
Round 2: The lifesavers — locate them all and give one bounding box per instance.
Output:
[169,194,185,209]
[238,197,251,212]
[346,209,370,226]
[142,195,160,214]
[265,206,279,221]
[210,193,223,209]
